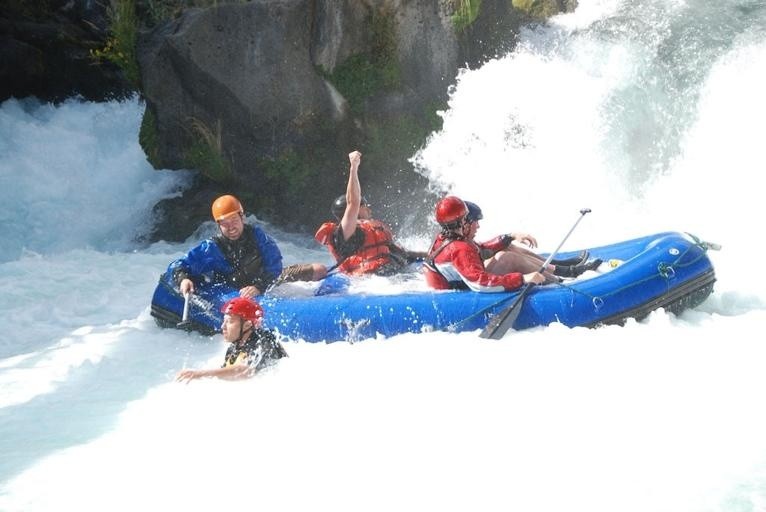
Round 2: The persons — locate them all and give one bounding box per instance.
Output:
[463,200,604,278]
[176,295,287,383]
[166,195,330,302]
[424,196,564,295]
[315,149,429,276]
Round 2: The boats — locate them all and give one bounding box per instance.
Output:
[151,231,715,339]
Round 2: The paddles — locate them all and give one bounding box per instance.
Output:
[479,209,592,341]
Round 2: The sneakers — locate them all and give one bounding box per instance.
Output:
[569,250,603,279]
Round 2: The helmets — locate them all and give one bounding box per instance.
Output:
[211,195,243,222]
[221,297,264,320]
[330,194,367,221]
[435,196,484,223]
